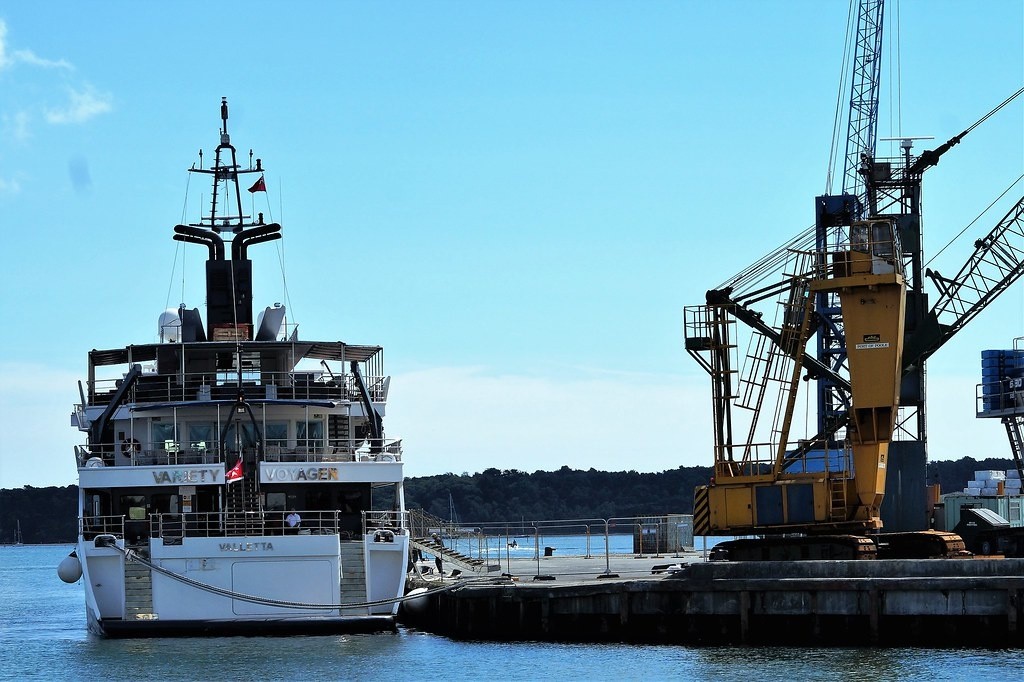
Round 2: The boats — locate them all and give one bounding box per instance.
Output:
[71,96,412,637]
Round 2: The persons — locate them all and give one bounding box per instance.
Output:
[286,507,301,535]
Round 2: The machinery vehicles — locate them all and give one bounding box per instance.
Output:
[685,1,1024,555]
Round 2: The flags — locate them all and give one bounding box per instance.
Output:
[226,459,244,484]
[247,176,266,193]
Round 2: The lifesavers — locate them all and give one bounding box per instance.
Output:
[123,437,141,458]
